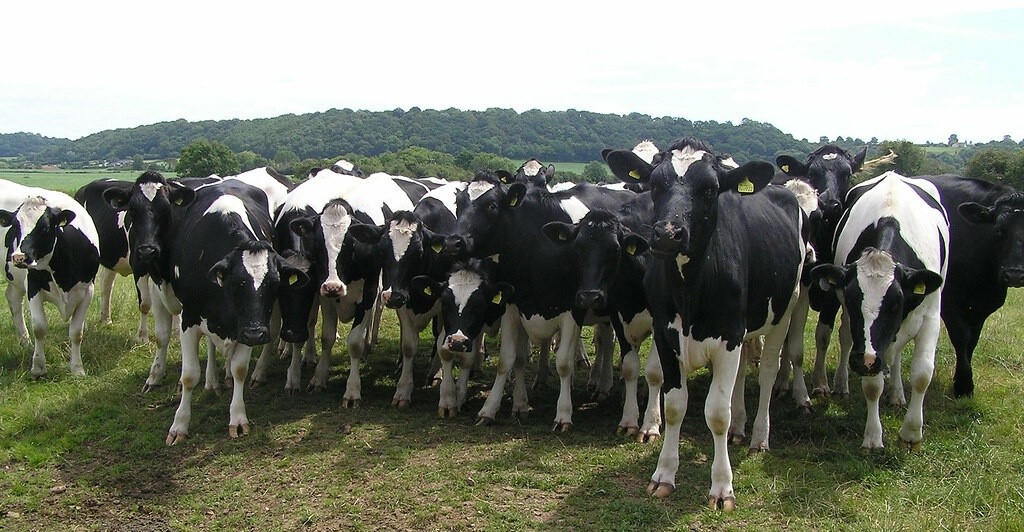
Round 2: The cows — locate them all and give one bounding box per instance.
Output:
[1,134,1024,518]
